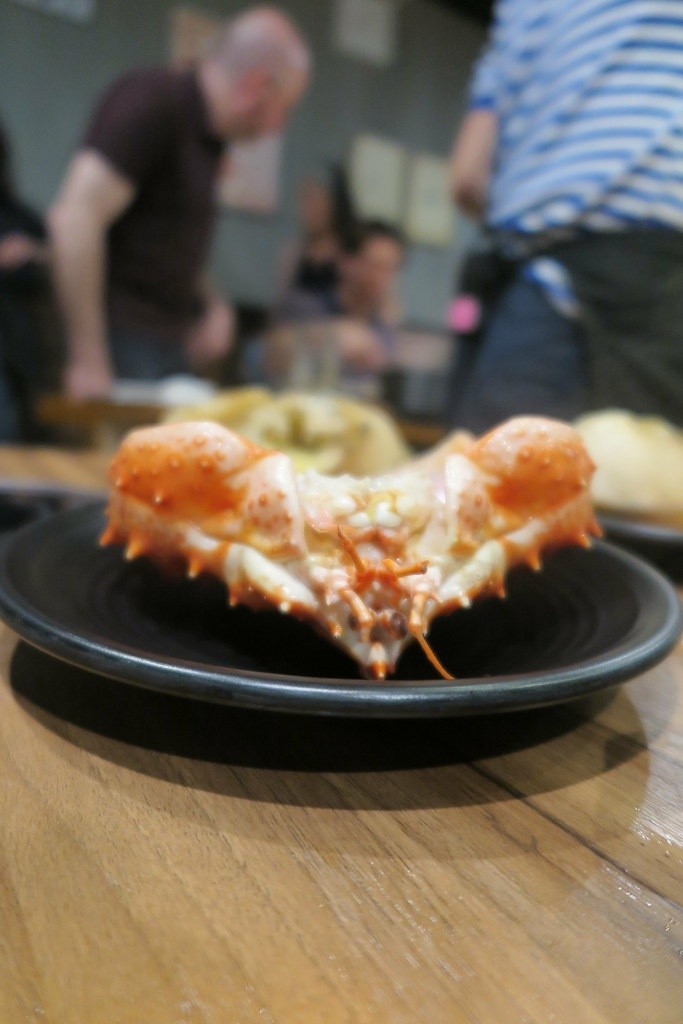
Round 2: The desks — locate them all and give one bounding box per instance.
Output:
[1,444,683,1024]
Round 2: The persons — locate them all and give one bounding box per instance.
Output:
[0,11,418,421]
[453,0,683,440]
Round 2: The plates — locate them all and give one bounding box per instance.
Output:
[0,495,683,774]
[583,506,683,587]
